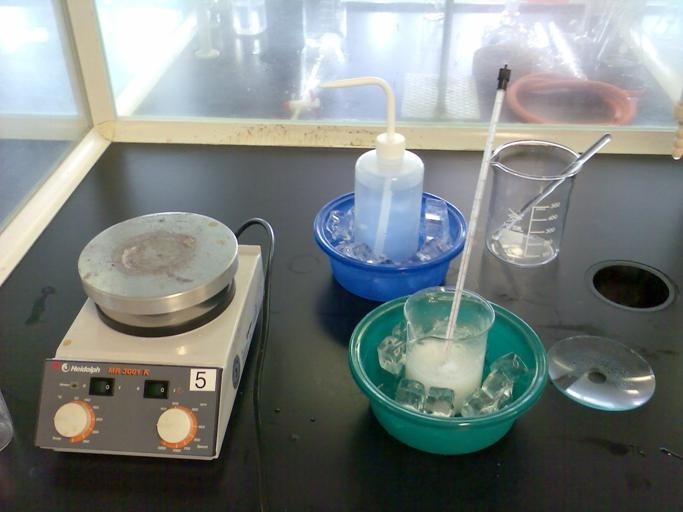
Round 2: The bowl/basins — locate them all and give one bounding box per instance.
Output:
[347,291,549,455]
[312,187,468,302]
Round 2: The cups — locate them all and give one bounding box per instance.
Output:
[484,137,584,269]
[402,284,496,417]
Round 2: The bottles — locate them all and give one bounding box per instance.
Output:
[352,131,425,262]
